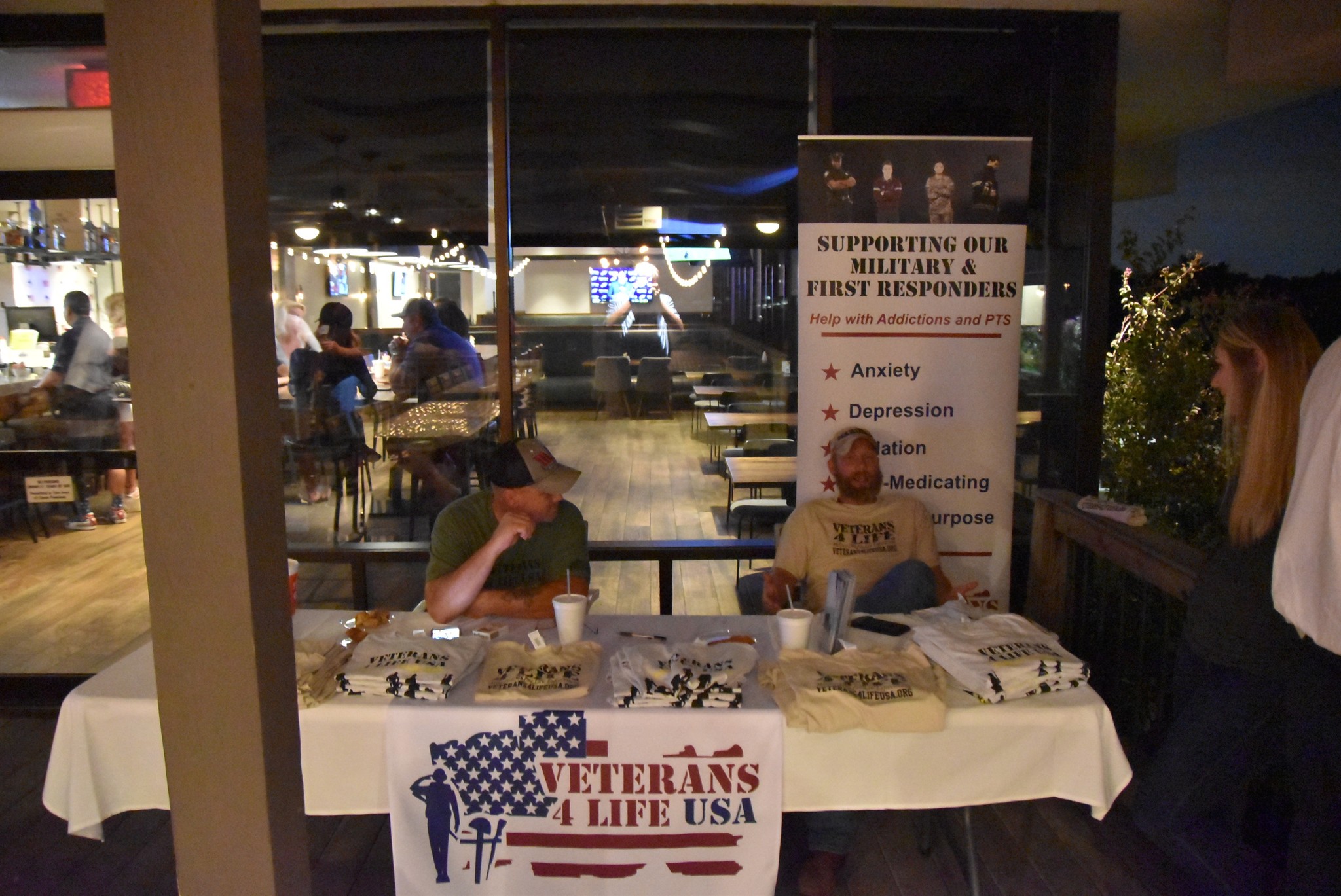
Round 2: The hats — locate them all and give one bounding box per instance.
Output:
[488,438,582,493]
[391,298,437,319]
[314,302,354,329]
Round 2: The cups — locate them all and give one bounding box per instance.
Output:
[287,559,299,616]
[776,609,814,652]
[552,594,587,644]
[371,359,385,378]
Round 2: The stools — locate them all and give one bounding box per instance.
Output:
[285,435,359,532]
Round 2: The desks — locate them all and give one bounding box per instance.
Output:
[0,418,138,543]
[684,371,759,379]
[704,413,798,473]
[279,344,543,518]
[40,608,1133,896]
[693,385,787,414]
[725,456,798,527]
[582,359,642,366]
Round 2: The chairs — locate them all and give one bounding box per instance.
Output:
[736,571,771,614]
[594,356,798,590]
[512,360,539,435]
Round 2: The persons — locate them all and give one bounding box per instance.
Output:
[26,290,137,532]
[1126,302,1341,896]
[873,161,902,223]
[761,426,981,896]
[970,154,1001,224]
[925,162,955,224]
[604,261,684,375]
[420,436,591,625]
[271,297,382,505]
[386,296,487,515]
[822,151,859,222]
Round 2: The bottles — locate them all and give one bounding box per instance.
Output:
[2,218,112,253]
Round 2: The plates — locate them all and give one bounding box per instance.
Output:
[696,629,757,647]
[343,614,395,633]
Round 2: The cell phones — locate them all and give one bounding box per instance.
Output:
[851,615,912,636]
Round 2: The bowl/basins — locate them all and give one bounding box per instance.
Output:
[12,368,31,378]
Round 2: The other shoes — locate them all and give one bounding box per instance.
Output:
[104,502,126,523]
[125,486,141,503]
[64,512,97,531]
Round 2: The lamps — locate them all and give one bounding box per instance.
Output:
[313,135,490,273]
